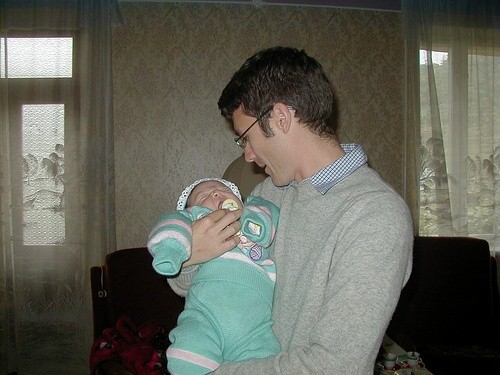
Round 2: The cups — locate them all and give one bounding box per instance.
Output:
[383,353,397,370]
[407,352,422,367]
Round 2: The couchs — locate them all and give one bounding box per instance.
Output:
[88,235,499,374]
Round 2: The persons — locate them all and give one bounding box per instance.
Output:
[145,176,288,375]
[165,45,414,374]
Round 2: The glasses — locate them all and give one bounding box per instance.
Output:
[234,107,274,149]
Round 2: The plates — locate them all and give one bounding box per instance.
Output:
[400,360,424,369]
[376,363,401,371]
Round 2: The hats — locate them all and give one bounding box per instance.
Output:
[174,176,243,210]
